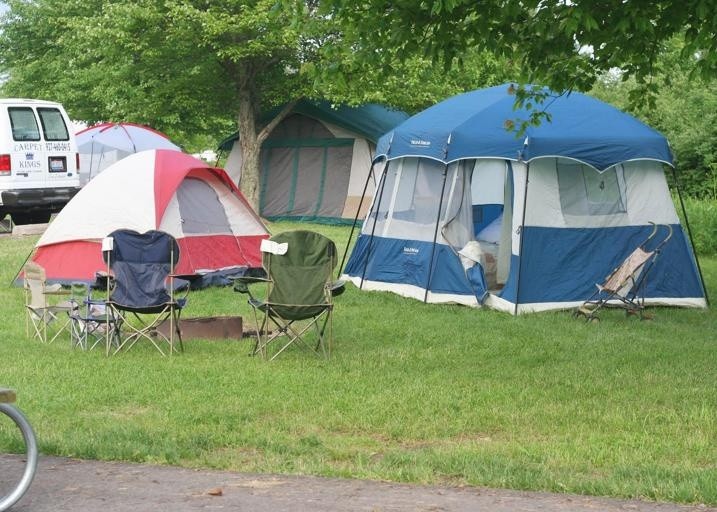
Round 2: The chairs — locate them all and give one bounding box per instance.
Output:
[101,228,191,358]
[67,283,126,352]
[227,229,345,363]
[23,262,80,348]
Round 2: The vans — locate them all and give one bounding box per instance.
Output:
[0,98,81,223]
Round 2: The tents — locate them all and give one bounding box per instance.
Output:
[12,148,274,289]
[220,94,410,227]
[75,122,182,193]
[339,81,708,316]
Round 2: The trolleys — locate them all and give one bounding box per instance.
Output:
[571,221,674,327]
